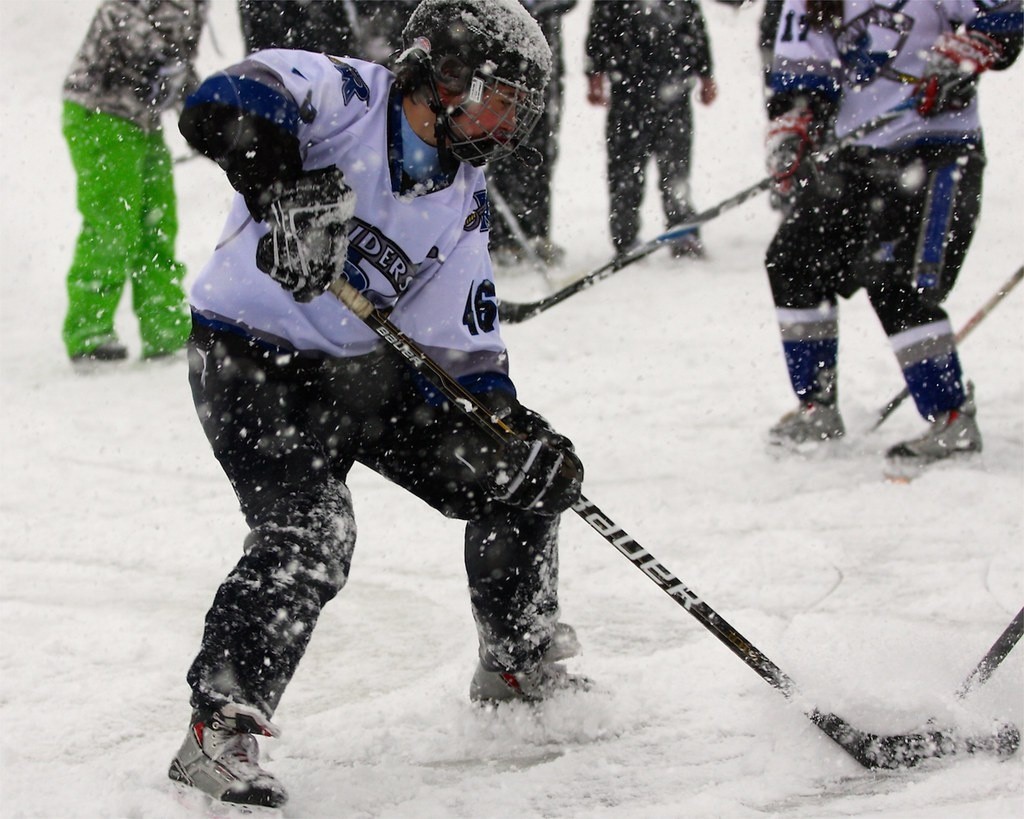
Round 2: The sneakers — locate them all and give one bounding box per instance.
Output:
[470,622,596,705]
[72,343,127,367]
[166,695,288,819]
[768,400,845,445]
[883,379,982,467]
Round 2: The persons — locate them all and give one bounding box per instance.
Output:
[62,0,1023,480]
[167,0,581,816]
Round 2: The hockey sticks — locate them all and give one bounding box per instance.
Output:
[862,251,1023,443]
[899,599,1023,734]
[323,272,1024,780]
[483,173,566,293]
[492,88,921,324]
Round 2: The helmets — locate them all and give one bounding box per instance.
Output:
[401,0,551,167]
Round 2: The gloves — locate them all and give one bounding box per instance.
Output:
[451,386,585,516]
[909,28,1005,120]
[255,164,357,304]
[763,92,825,209]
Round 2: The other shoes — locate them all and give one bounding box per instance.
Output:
[489,235,567,277]
[670,239,704,259]
